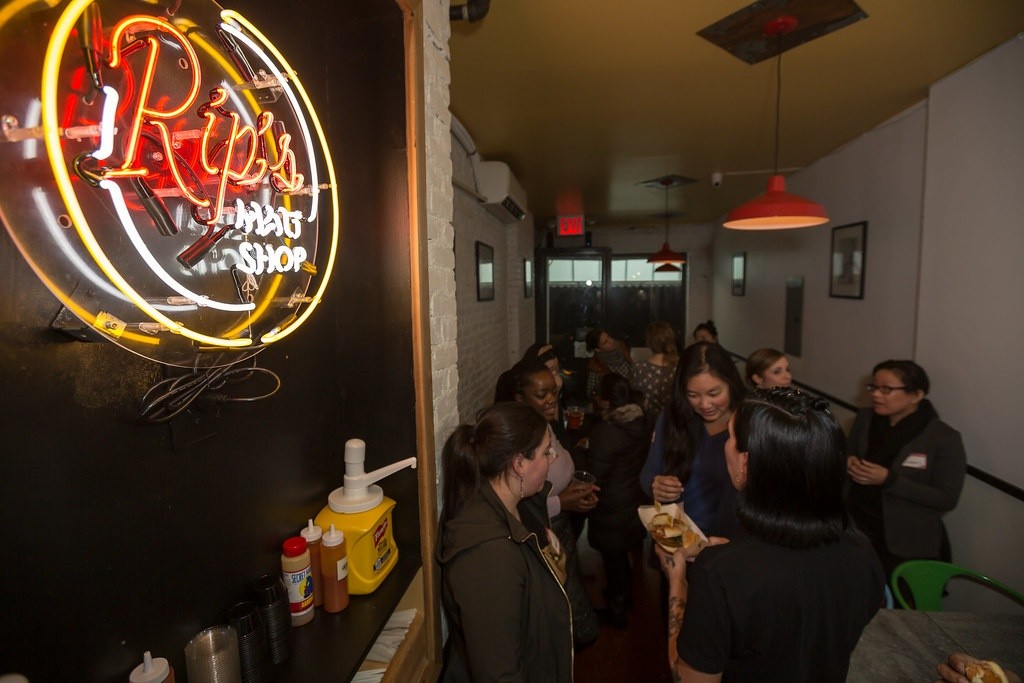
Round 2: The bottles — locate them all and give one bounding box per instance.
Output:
[319,524,349,613]
[281,537,315,627]
[300,519,323,607]
[129,651,175,683]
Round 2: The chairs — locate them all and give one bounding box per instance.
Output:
[892,560,1023,612]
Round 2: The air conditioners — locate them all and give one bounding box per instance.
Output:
[478,159,530,223]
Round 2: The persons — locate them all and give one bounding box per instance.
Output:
[693,320,718,344]
[525,341,589,545]
[585,327,633,378]
[627,322,681,406]
[434,399,577,683]
[583,374,657,625]
[494,361,601,651]
[745,347,792,389]
[638,340,886,683]
[836,359,967,610]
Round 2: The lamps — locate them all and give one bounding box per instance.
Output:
[719,17,829,229]
[646,179,686,276]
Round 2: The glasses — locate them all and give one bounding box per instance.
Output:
[865,383,906,394]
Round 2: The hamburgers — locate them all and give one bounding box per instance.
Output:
[651,512,701,552]
[965,659,1009,683]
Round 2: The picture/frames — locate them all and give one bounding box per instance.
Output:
[728,255,747,297]
[832,222,865,298]
[475,239,495,301]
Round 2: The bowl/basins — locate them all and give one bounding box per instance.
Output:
[185,575,294,683]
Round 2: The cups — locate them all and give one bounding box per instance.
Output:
[569,470,596,502]
[566,406,584,430]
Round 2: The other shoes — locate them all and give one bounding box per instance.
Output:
[593,607,628,631]
[603,586,634,609]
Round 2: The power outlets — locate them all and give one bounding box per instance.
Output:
[164,351,227,454]
